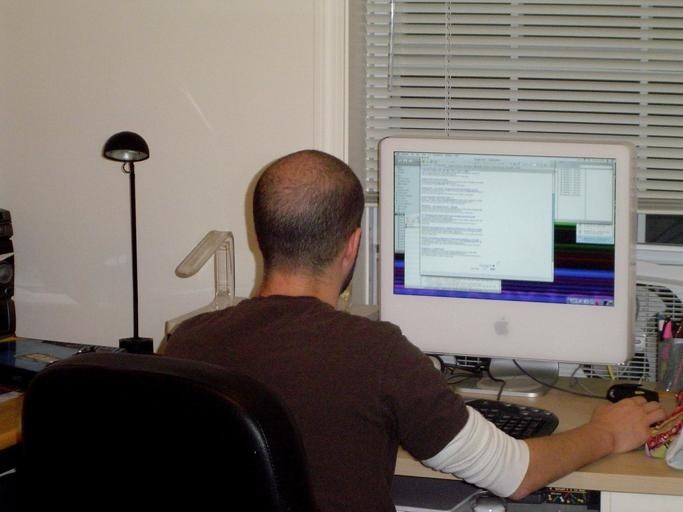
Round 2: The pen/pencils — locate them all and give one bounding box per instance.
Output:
[658,319,683,392]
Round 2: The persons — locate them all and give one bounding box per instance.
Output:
[151,148,671,512]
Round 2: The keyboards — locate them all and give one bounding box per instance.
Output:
[464,398,561,439]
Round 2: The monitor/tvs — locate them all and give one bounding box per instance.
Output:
[378,135,638,399]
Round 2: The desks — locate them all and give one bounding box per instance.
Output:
[384,365,682,512]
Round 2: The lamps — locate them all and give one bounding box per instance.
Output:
[164,226,249,337]
[102,128,152,362]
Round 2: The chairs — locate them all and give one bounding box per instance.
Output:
[13,353,325,512]
